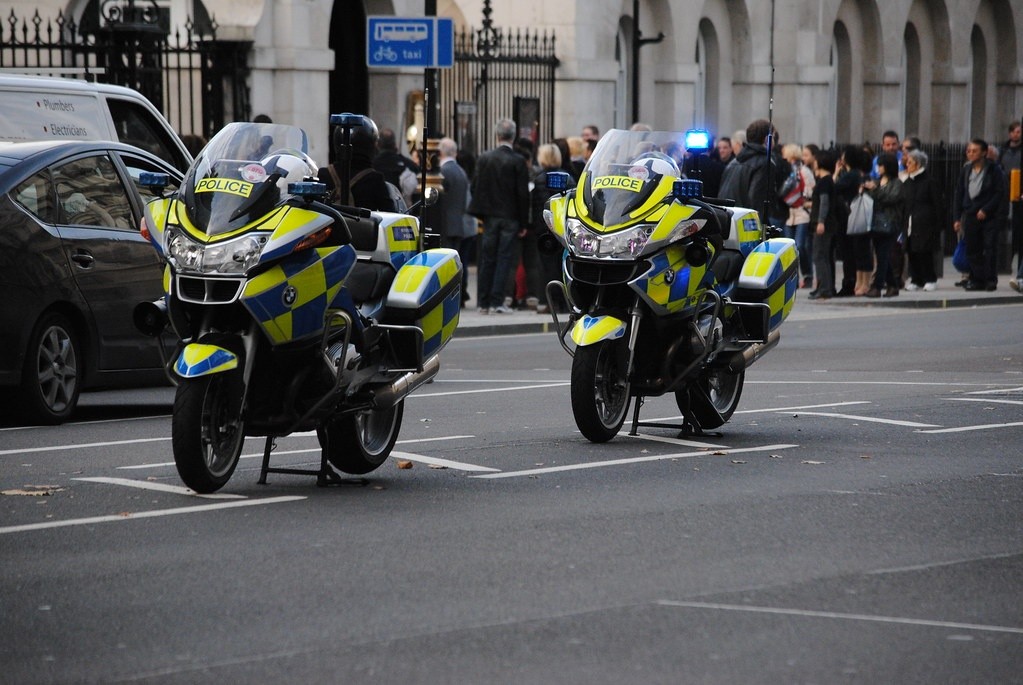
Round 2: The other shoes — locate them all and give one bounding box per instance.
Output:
[1010,278,1023,293]
[865,287,882,297]
[905,284,919,291]
[475,296,572,315]
[809,286,836,298]
[883,287,899,297]
[922,281,936,291]
[955,279,997,290]
[801,278,812,288]
[834,289,855,297]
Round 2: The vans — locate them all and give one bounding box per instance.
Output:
[0,71,192,171]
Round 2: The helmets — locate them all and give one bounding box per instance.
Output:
[631,150,680,180]
[260,148,318,200]
[332,114,380,154]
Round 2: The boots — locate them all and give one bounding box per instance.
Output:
[853,270,873,296]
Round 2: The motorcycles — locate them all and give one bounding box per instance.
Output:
[541,127,800,444]
[145,122,463,494]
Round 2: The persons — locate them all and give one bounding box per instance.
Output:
[722,120,790,233]
[378,130,417,206]
[629,123,745,194]
[430,119,600,313]
[953,121,1023,292]
[773,131,944,298]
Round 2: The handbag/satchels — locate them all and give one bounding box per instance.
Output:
[1010,168,1021,202]
[846,193,874,235]
[953,238,971,274]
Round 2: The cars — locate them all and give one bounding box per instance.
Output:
[0,140,198,425]
